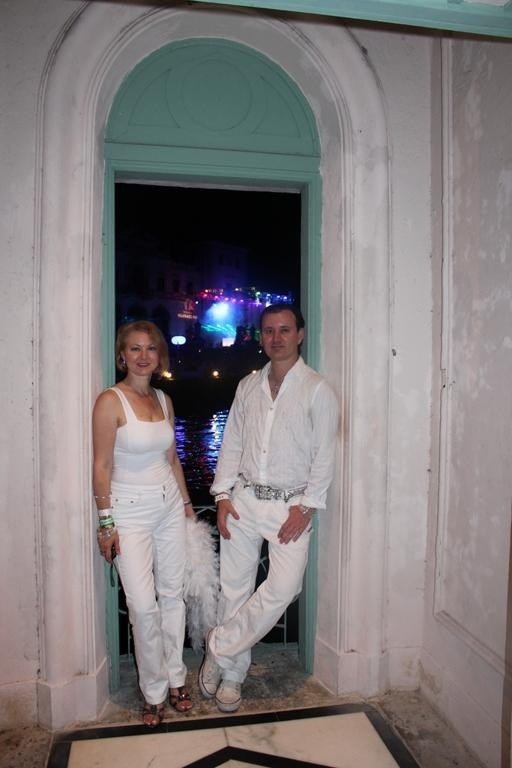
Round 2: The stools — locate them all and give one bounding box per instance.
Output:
[240,471,308,503]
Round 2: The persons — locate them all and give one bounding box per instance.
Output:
[91,320,197,728]
[198,303,339,714]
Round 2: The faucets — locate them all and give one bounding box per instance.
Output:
[142,700,166,728]
[168,683,194,711]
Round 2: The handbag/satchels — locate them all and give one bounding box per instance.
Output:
[94,492,115,541]
[184,500,192,505]
[214,494,230,506]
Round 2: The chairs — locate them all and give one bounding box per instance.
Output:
[214,676,244,712]
[198,625,221,698]
